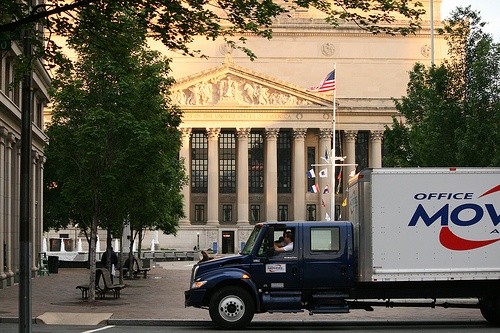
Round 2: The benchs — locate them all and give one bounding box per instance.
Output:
[99,268,126,299]
[134,257,150,279]
[76,269,103,299]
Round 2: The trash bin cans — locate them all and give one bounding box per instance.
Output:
[48,256,59,273]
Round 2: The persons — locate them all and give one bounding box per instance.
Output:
[274,231,293,252]
[101,248,135,285]
[199,251,209,262]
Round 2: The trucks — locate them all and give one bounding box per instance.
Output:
[185,167,500,327]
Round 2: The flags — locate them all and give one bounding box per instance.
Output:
[306,68,336,92]
[306,169,355,221]
